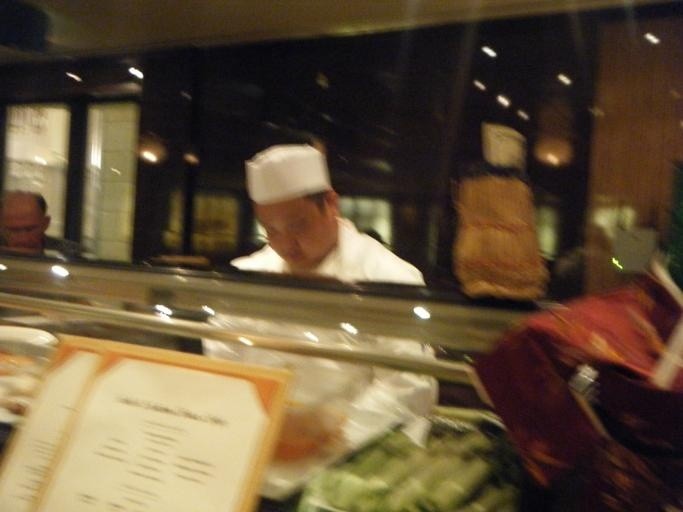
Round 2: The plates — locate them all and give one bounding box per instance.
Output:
[258,398,398,500]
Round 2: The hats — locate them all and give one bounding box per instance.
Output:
[244,141,332,206]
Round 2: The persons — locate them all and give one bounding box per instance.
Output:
[0,188,49,249]
[199,143,435,392]
